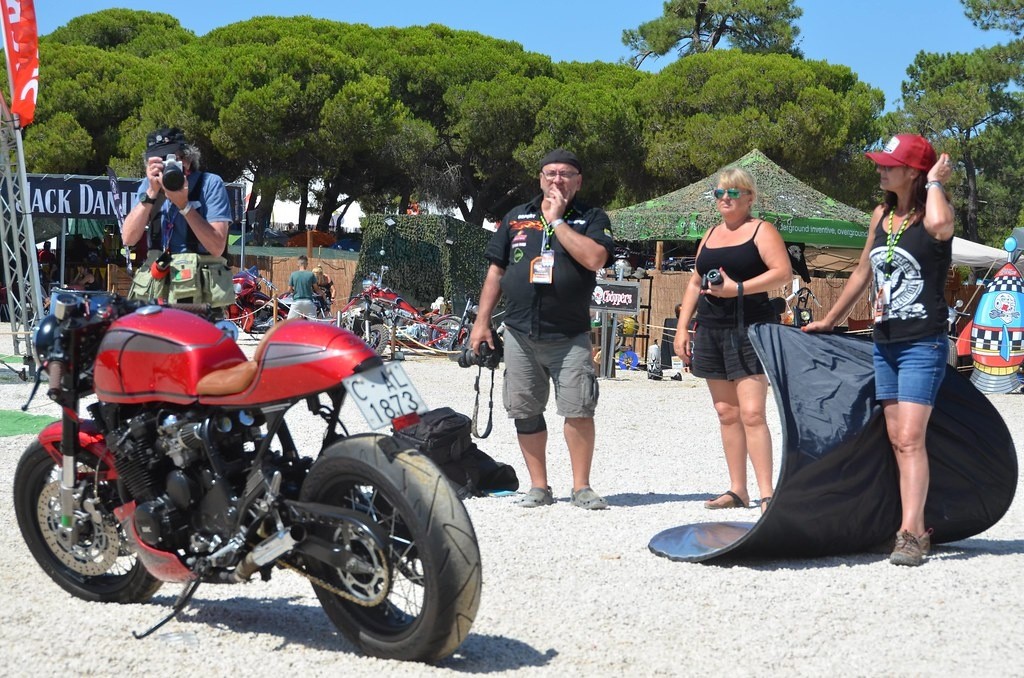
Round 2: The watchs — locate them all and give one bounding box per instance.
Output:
[139,192,156,204]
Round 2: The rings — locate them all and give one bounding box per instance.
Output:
[185,187,188,189]
[944,162,949,165]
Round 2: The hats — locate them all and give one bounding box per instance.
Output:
[145,127,185,161]
[865,134,937,172]
[540,150,583,173]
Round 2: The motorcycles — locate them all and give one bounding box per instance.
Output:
[213,263,495,360]
[946,300,972,368]
[11,249,484,664]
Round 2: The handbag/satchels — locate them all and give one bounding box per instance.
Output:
[393,407,520,500]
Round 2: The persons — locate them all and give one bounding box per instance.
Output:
[122,128,234,257]
[40,237,103,291]
[288,254,326,321]
[470,152,617,508]
[673,166,793,514]
[802,135,956,566]
[312,265,337,317]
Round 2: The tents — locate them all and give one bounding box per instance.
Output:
[606,150,872,247]
[233,228,363,252]
[1008,226,1024,264]
[950,237,1024,268]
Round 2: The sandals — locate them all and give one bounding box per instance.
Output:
[571,487,608,509]
[520,486,553,507]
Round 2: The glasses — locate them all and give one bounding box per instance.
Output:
[714,188,751,199]
[541,170,581,179]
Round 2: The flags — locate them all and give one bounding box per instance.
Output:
[1,0,39,127]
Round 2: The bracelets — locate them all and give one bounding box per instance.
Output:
[925,180,942,190]
[179,202,191,215]
[551,219,563,228]
[738,282,743,296]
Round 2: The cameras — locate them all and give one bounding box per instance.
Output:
[701,269,723,291]
[158,154,185,192]
[457,328,503,368]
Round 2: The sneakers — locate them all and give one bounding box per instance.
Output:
[875,528,934,554]
[890,529,923,565]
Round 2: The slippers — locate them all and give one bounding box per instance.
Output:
[761,497,771,516]
[704,491,749,509]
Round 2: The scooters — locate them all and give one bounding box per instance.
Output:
[784,286,824,329]
